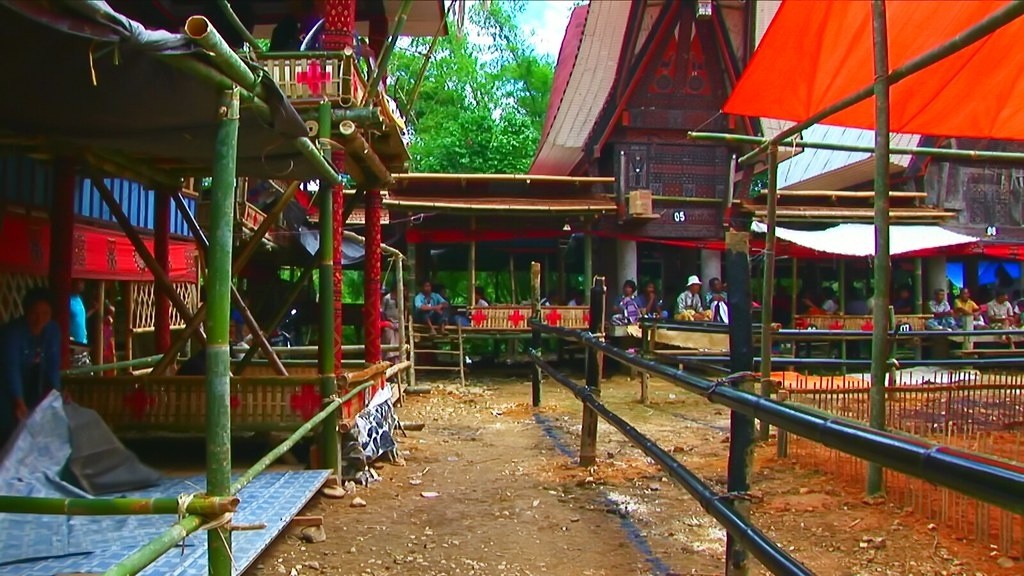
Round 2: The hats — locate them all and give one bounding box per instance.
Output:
[686,275,703,286]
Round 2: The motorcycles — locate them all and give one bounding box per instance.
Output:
[266,309,297,348]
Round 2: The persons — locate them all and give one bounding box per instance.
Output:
[540,290,585,307]
[379,280,492,384]
[609,275,728,357]
[924,287,1024,350]
[0,278,118,452]
[176,285,255,376]
[750,273,916,330]
